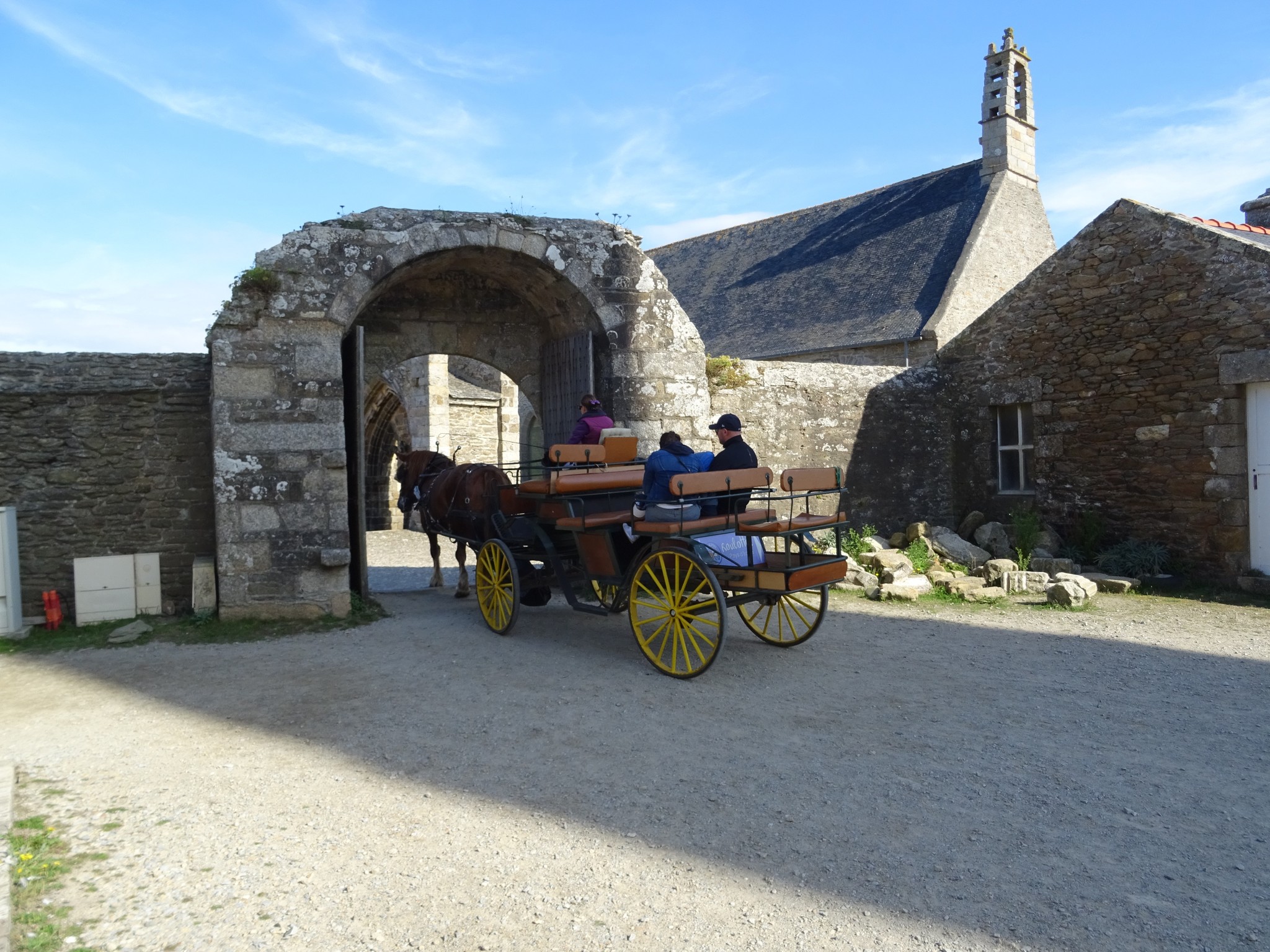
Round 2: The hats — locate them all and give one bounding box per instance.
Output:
[709,413,741,432]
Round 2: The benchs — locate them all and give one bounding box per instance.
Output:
[735,464,846,567]
[557,467,650,531]
[635,466,778,561]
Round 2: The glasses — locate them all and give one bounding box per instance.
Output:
[715,429,719,434]
[578,405,582,410]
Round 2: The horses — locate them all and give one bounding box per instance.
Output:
[390,442,519,597]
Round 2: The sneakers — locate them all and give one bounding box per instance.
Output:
[623,523,639,544]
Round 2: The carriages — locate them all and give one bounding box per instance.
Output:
[394,429,842,679]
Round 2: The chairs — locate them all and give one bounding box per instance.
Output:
[595,428,637,467]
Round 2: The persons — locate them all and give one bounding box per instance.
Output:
[540,394,614,480]
[701,413,758,514]
[623,431,715,545]
